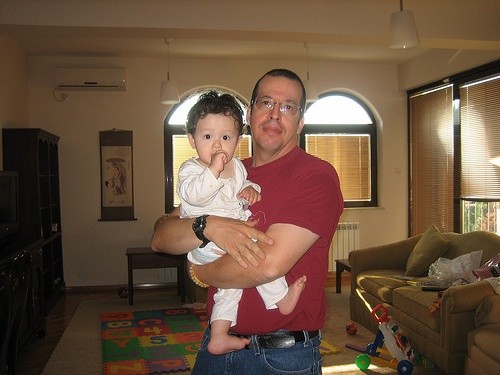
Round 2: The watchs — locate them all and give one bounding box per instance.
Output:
[192,214,210,249]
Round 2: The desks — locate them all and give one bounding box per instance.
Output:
[126,247,190,305]
[334,259,352,294]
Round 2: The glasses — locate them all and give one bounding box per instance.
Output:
[252,96,302,116]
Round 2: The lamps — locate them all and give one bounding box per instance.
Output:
[388,0,419,50]
[303,42,319,102]
[158,36,180,105]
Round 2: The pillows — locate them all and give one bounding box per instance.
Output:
[404,223,451,278]
[451,250,484,286]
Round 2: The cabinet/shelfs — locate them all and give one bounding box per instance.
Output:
[0,127,66,375]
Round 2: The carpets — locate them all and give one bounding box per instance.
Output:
[38,291,448,375]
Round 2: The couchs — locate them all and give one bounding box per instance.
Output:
[347,230,500,375]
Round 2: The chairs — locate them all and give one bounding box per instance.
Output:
[0,271,26,375]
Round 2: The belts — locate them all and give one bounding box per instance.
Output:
[209,325,320,349]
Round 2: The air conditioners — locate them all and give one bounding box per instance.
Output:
[52,67,127,102]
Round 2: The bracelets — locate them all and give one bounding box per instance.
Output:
[189,263,209,288]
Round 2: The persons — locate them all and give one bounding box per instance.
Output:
[151,69,345,375]
[176,88,307,355]
